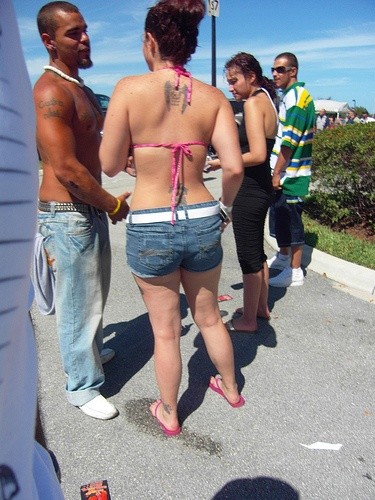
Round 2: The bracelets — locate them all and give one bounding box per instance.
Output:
[108,196,120,215]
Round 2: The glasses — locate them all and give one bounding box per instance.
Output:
[271,66,294,73]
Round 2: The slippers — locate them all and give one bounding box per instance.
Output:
[208,374,245,407]
[148,398,181,435]
[236,308,271,320]
[224,319,257,334]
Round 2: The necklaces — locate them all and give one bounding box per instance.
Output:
[42,65,85,88]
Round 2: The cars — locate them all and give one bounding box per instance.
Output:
[94,94,111,118]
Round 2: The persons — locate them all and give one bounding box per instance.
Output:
[316,108,375,131]
[203,51,279,334]
[32,0,131,419]
[99,0,245,437]
[265,53,315,287]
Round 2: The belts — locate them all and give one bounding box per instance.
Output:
[39,201,107,217]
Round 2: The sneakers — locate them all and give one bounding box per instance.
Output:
[268,266,305,287]
[100,348,115,364]
[266,255,291,270]
[79,395,118,420]
